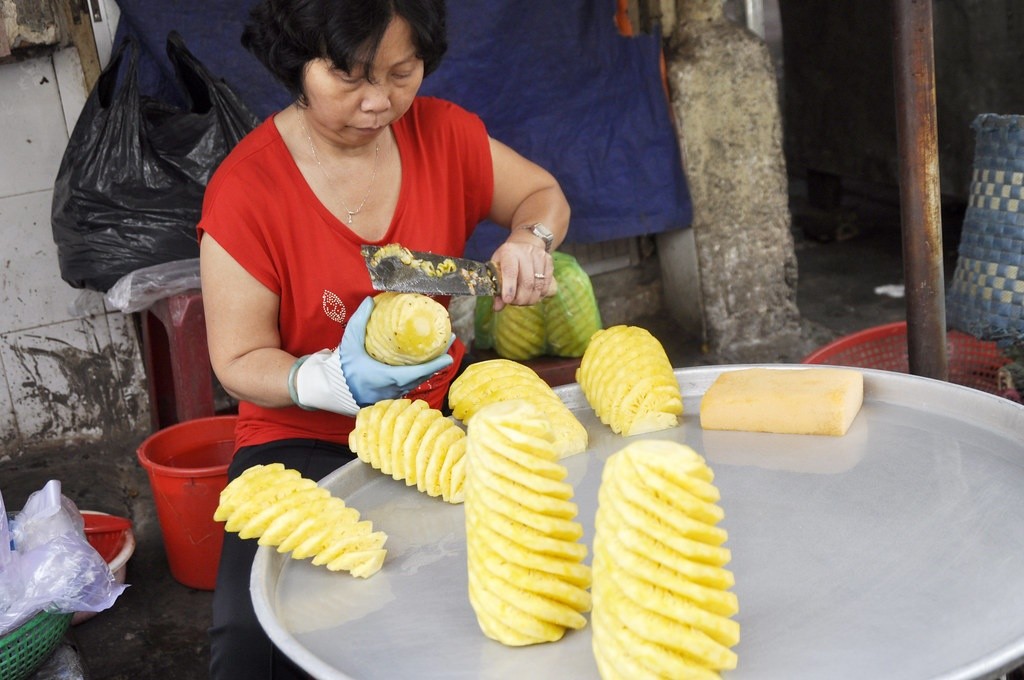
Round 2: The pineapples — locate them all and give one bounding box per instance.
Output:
[214,326,685,576]
[589,440,741,680]
[362,250,602,365]
[463,402,589,647]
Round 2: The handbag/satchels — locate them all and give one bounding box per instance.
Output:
[52,26,268,290]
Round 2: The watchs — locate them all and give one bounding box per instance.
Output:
[508,222,555,254]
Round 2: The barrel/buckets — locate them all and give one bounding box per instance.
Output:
[137,414,239,591]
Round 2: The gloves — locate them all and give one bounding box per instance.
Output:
[295,295,458,418]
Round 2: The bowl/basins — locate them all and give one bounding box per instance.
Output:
[72,509,135,625]
[81,514,133,564]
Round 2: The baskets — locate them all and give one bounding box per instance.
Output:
[804,320,1022,403]
[946,112,1024,342]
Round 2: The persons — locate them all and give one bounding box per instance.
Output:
[194,0,574,680]
